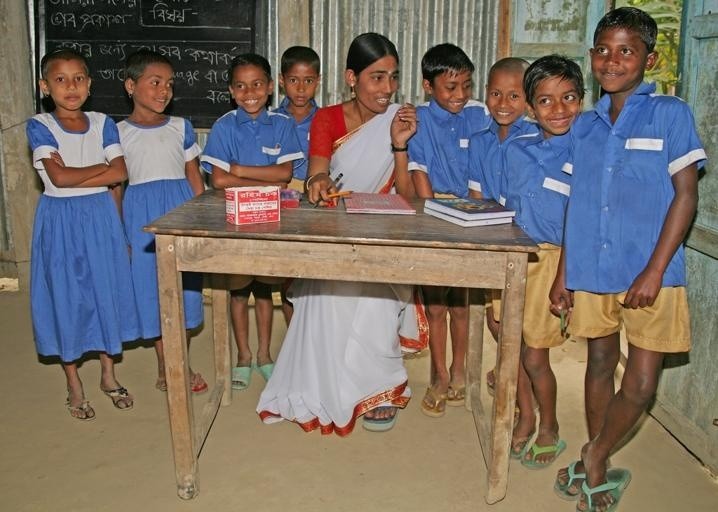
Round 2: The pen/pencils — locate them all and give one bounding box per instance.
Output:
[311,173,343,209]
[320,191,353,199]
[560,308,565,337]
[564,308,572,333]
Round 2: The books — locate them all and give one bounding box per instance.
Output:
[341,192,417,216]
[423,206,513,228]
[425,198,516,221]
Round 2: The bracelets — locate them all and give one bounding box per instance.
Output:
[391,143,410,157]
[305,173,315,191]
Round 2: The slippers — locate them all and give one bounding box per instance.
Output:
[189,372,208,395]
[487,365,495,397]
[230,364,251,391]
[520,439,566,470]
[513,400,539,428]
[420,386,447,418]
[155,376,167,392]
[66,390,96,422]
[361,406,400,433]
[553,458,610,502]
[509,426,534,459]
[576,468,631,512]
[99,381,133,412]
[444,382,466,406]
[253,362,273,383]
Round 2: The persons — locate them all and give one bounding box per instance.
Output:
[198,54,305,388]
[467,53,539,398]
[114,51,207,394]
[256,32,431,436]
[25,47,135,423]
[404,45,489,417]
[507,56,587,468]
[269,44,321,325]
[549,6,709,512]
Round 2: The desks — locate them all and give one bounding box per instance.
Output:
[142,188,541,504]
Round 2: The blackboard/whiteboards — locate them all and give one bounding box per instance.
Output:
[26,0,279,135]
